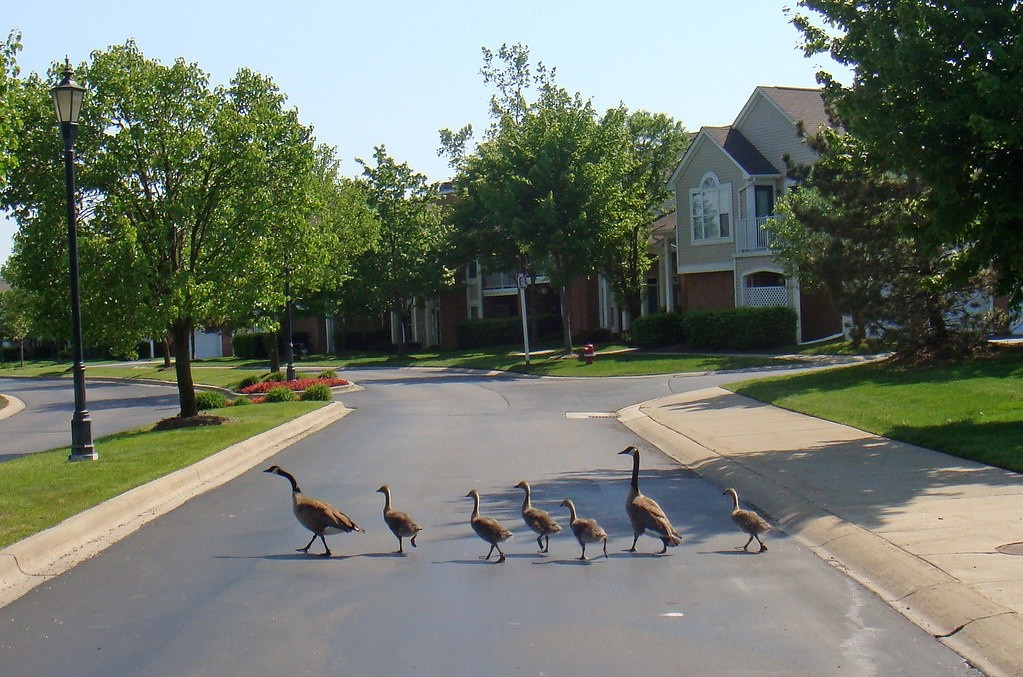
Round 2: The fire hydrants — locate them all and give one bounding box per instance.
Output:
[582,343,596,364]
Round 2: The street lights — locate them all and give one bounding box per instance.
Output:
[47,54,99,462]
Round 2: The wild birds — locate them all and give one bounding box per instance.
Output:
[559,499,608,561]
[262,465,366,558]
[465,490,513,563]
[514,481,563,553]
[618,446,682,554]
[376,485,423,554]
[723,487,772,553]
